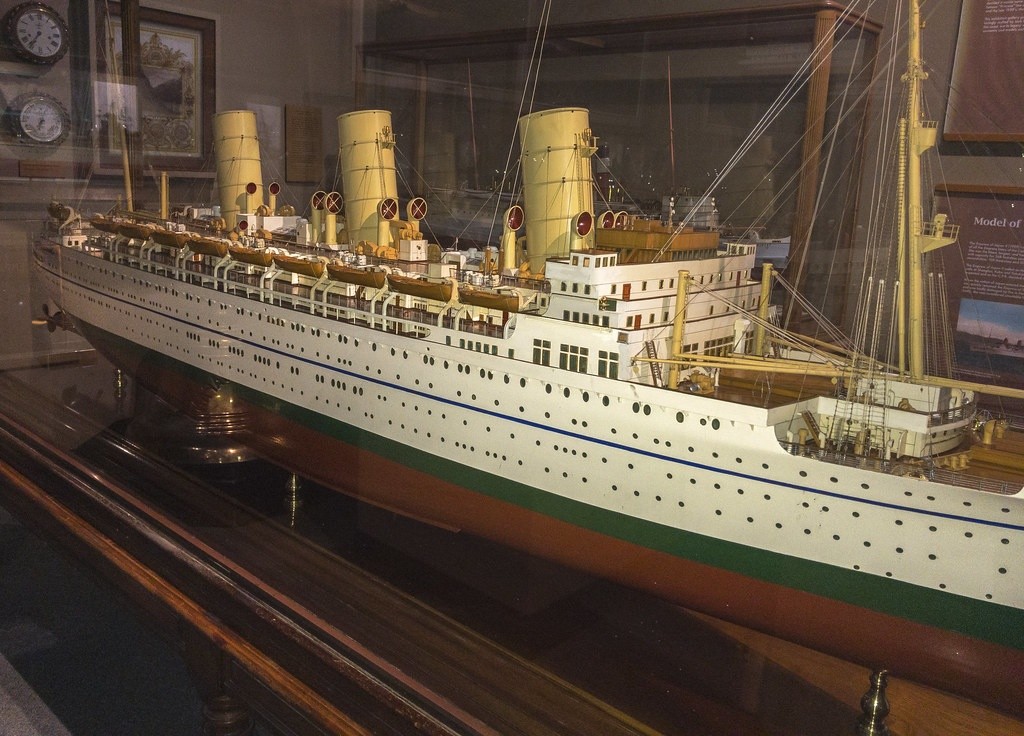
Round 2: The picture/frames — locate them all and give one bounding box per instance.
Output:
[80,0,217,181]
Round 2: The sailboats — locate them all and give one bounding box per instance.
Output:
[25,0,1022,722]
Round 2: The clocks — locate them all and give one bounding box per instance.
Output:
[4,1,71,66]
[3,92,71,151]
